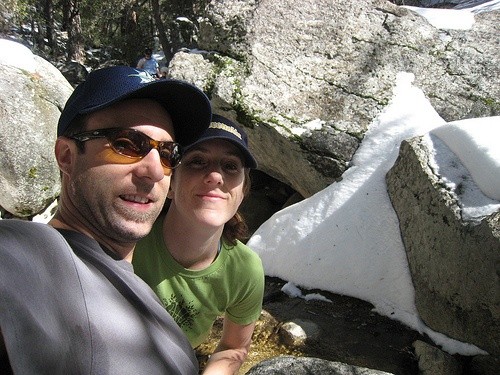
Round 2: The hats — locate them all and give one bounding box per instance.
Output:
[146,49,152,55]
[57,67,212,148]
[181,114,258,170]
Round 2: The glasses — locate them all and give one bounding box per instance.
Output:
[69,127,182,169]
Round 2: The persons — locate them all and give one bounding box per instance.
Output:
[137,48,162,79]
[160,71,167,80]
[0,65,212,375]
[129,113,265,375]
